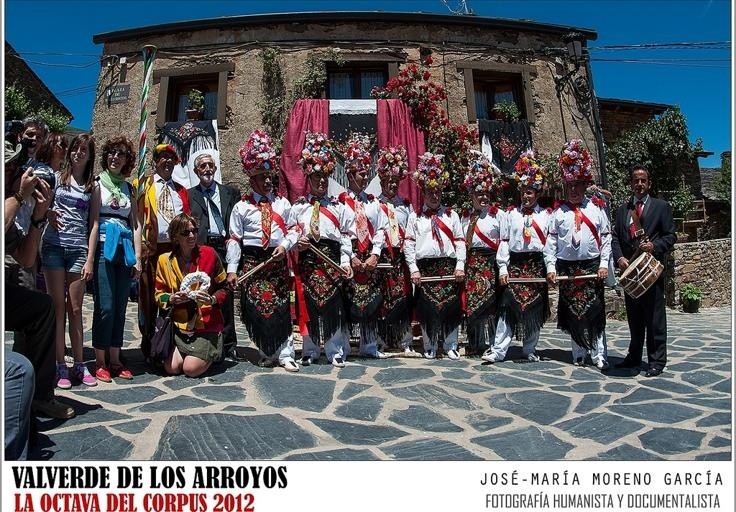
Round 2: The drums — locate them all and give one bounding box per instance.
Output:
[618,252,664,299]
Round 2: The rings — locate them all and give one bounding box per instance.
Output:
[204,296,208,301]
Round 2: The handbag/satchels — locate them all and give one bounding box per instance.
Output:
[139,318,173,366]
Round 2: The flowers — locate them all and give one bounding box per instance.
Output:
[463,209,468,216]
[241,194,251,202]
[367,193,373,202]
[415,207,420,214]
[375,144,408,181]
[338,191,347,205]
[295,192,307,204]
[276,189,285,199]
[592,195,603,208]
[410,152,449,190]
[295,130,335,174]
[336,124,377,173]
[328,196,337,205]
[557,139,593,183]
[546,206,552,212]
[238,129,277,175]
[506,204,514,211]
[512,148,550,191]
[553,200,559,208]
[445,207,451,214]
[459,153,509,192]
[490,202,500,214]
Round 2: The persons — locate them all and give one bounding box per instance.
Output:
[374,177,422,363]
[132,143,191,369]
[153,213,233,378]
[288,172,353,371]
[225,172,302,372]
[11,115,52,295]
[457,190,506,356]
[401,187,466,360]
[584,180,621,291]
[335,170,390,364]
[34,132,68,357]
[479,186,550,368]
[3,139,75,420]
[3,350,36,461]
[89,135,141,383]
[611,165,677,379]
[39,133,101,389]
[186,153,247,362]
[542,181,612,370]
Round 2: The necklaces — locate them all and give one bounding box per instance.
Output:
[178,254,190,267]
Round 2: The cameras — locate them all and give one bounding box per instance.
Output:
[30,173,55,189]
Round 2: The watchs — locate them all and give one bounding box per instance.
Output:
[29,214,47,228]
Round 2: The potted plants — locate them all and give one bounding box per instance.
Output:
[184,88,205,121]
[493,99,520,121]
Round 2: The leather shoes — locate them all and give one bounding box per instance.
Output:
[645,367,665,377]
[614,359,642,367]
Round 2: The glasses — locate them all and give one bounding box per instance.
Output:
[179,228,199,235]
[109,150,125,158]
[163,154,175,162]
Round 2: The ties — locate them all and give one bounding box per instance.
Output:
[158,182,175,220]
[249,196,594,253]
[204,189,227,237]
[630,200,644,236]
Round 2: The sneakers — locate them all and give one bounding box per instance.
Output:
[56,365,72,388]
[252,334,611,373]
[110,363,132,379]
[93,364,112,383]
[72,364,97,386]
[35,398,76,419]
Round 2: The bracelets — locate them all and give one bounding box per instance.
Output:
[15,192,26,208]
[210,296,215,305]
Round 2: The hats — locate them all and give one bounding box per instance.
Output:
[4,141,22,168]
